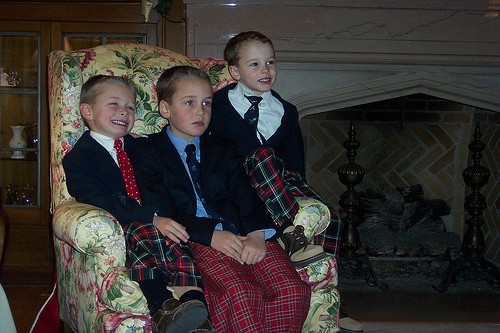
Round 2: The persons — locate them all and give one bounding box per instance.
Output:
[128,65,312,333]
[211,31,363,333]
[60,75,212,333]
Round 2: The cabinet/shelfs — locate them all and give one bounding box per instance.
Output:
[0,0,190,288]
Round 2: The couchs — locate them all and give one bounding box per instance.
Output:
[50,43,338,333]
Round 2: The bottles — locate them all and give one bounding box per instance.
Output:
[27,122,38,160]
[9,126,26,159]
[0,67,12,86]
[6,184,35,206]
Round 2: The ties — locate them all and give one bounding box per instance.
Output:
[242,94,263,137]
[184,144,239,235]
[113,138,141,201]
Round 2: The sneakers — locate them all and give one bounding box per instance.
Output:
[151,298,207,333]
[338,317,364,333]
[277,224,326,267]
